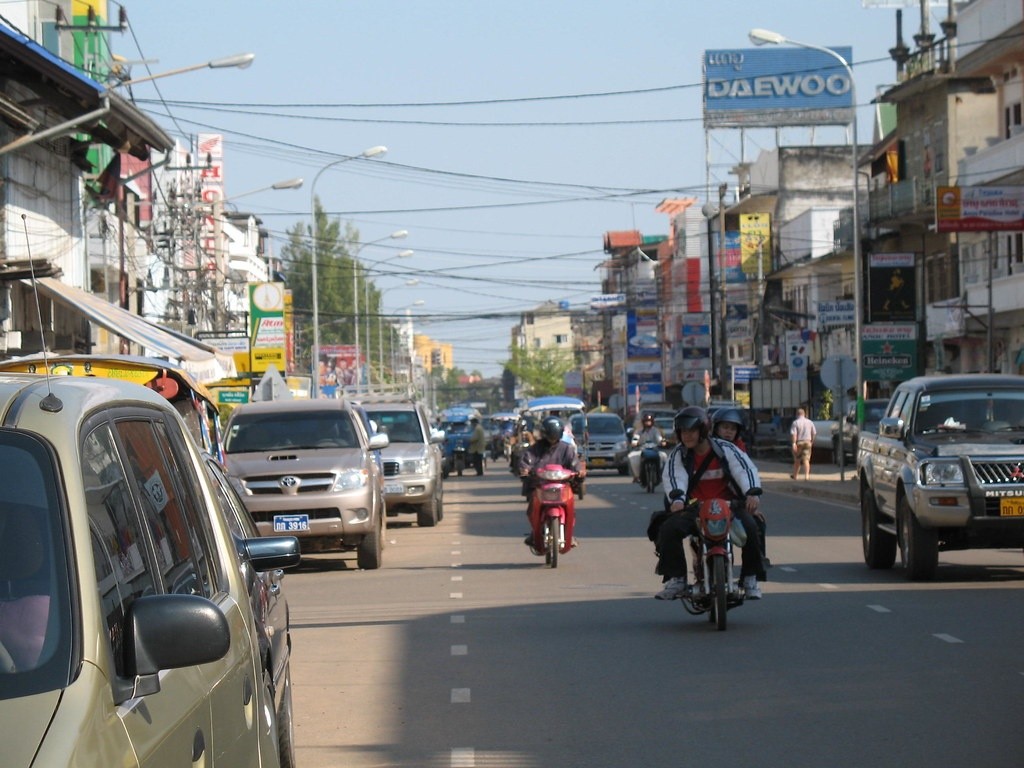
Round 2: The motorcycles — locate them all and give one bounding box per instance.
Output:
[653,487,767,632]
[633,437,669,492]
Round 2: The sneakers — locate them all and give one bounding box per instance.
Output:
[738,572,761,599]
[655,577,687,601]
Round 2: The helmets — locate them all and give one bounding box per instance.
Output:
[540,416,564,438]
[642,413,655,427]
[711,407,744,440]
[674,405,710,444]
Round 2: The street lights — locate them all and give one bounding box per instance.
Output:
[313,145,388,399]
[353,230,409,393]
[749,28,864,435]
[212,178,304,337]
[390,300,425,391]
[378,278,419,393]
[366,250,415,393]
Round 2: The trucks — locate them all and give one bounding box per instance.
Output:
[436,409,484,478]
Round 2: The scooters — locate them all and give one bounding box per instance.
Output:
[522,452,580,569]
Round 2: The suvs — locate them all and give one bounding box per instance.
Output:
[225,397,391,570]
[351,399,446,528]
[351,401,387,551]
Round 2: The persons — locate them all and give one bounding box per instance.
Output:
[510,418,537,478]
[521,416,586,549]
[0,500,51,671]
[655,407,768,601]
[468,416,486,477]
[790,407,816,480]
[627,410,667,484]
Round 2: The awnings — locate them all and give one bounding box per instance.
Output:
[0,12,175,154]
[16,269,220,359]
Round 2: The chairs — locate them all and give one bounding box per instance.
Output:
[0,503,50,671]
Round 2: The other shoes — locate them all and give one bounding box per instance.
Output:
[790,475,795,479]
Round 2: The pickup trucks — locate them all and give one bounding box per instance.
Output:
[856,372,1024,582]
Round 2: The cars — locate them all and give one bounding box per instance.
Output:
[0,372,282,768]
[569,412,635,476]
[655,418,678,448]
[195,448,302,768]
[830,398,891,468]
